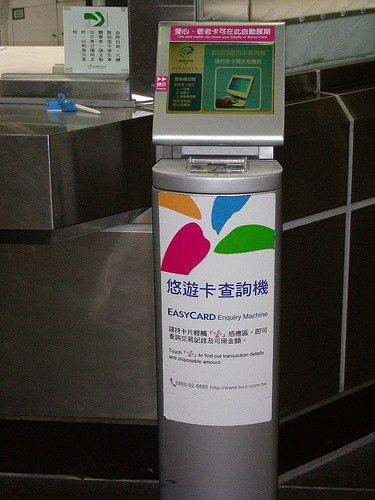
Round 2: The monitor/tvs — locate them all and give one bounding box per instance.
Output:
[152,20,285,146]
[225,74,255,99]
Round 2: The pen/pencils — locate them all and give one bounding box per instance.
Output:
[74,103,102,115]
